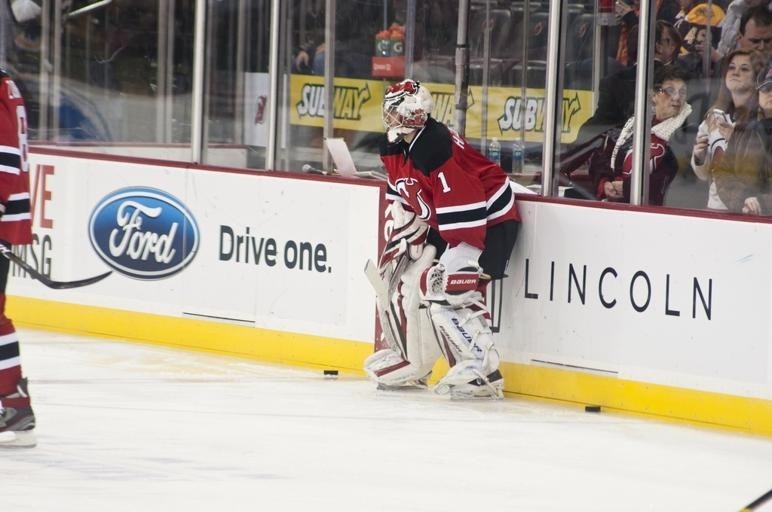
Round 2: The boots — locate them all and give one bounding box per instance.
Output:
[375,361,432,385]
[0,377,36,431]
[451,369,504,391]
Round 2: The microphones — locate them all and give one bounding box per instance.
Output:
[302,164,330,176]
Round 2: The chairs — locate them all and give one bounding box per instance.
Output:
[423,0,620,89]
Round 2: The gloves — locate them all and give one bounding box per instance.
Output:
[417,243,482,306]
[392,200,428,260]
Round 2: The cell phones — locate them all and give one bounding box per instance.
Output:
[713,109,728,123]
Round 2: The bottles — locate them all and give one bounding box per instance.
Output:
[489,137,501,167]
[511,137,525,180]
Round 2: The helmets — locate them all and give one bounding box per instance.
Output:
[381,79,435,144]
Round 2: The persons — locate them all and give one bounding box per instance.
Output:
[361,81,523,401]
[0,65,39,436]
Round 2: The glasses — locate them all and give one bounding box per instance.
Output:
[661,88,687,97]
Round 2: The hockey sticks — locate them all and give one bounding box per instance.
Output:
[0,243,111,289]
[364,252,411,311]
